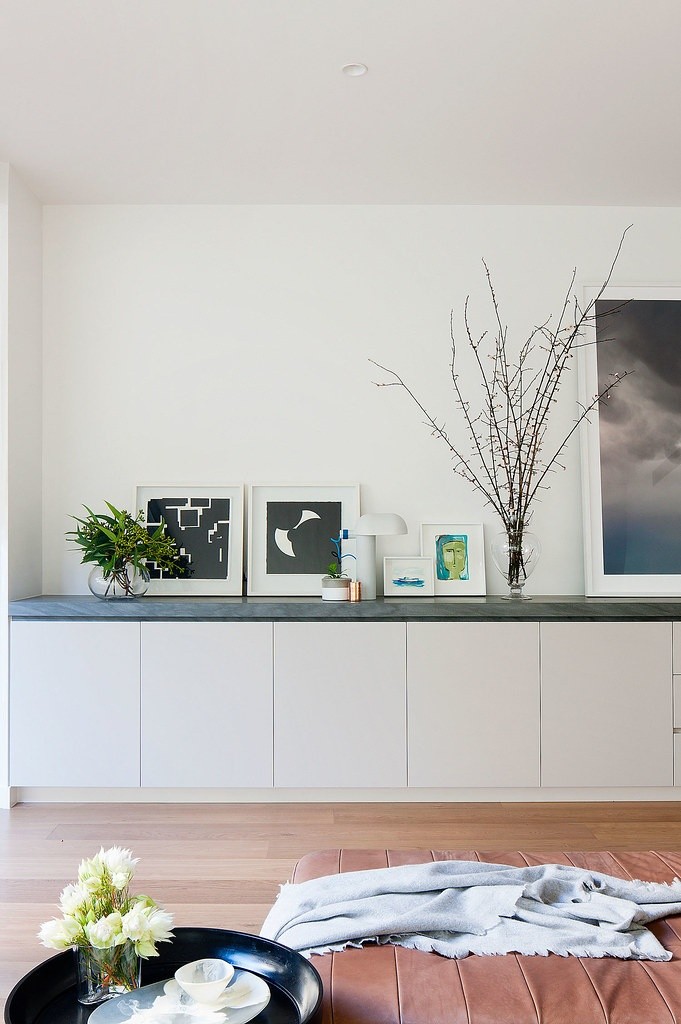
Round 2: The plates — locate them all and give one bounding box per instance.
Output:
[4,927,324,1024]
[86,969,271,1024]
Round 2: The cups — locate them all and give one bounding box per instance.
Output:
[174,958,234,1002]
[348,581,361,602]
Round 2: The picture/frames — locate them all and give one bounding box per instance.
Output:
[580,284,681,596]
[247,485,362,598]
[420,522,487,596]
[385,556,436,596]
[129,483,246,598]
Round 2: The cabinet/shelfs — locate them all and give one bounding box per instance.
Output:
[9,616,680,803]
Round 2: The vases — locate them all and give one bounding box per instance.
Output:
[79,933,148,1006]
[488,523,539,600]
[86,559,148,600]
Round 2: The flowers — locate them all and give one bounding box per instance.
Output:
[35,846,178,947]
[62,498,186,575]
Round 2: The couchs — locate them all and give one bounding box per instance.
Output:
[260,848,681,1024]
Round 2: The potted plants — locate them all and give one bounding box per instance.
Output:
[322,563,351,601]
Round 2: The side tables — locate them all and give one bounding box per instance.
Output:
[3,926,326,1024]
